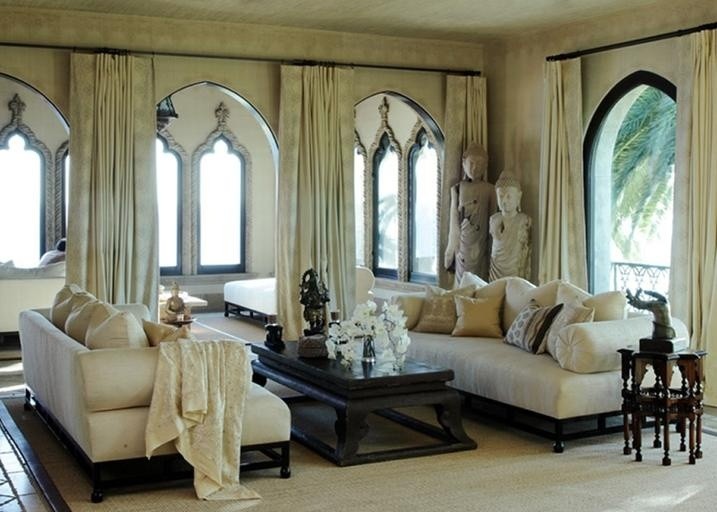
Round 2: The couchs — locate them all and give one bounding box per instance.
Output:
[16,302,294,502]
[0,260,66,337]
[388,289,693,454]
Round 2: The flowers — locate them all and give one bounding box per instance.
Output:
[324,299,411,372]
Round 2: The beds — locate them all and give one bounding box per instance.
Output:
[221,266,379,326]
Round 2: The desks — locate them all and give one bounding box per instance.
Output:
[615,343,710,467]
[182,296,208,331]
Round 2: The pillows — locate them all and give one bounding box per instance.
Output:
[49,282,199,350]
[411,271,629,363]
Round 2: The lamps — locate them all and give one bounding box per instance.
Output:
[156,96,181,134]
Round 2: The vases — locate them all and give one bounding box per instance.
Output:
[360,339,377,365]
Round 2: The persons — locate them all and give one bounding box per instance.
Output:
[38,237,67,266]
[444,142,498,288]
[488,171,532,283]
[165,280,185,322]
